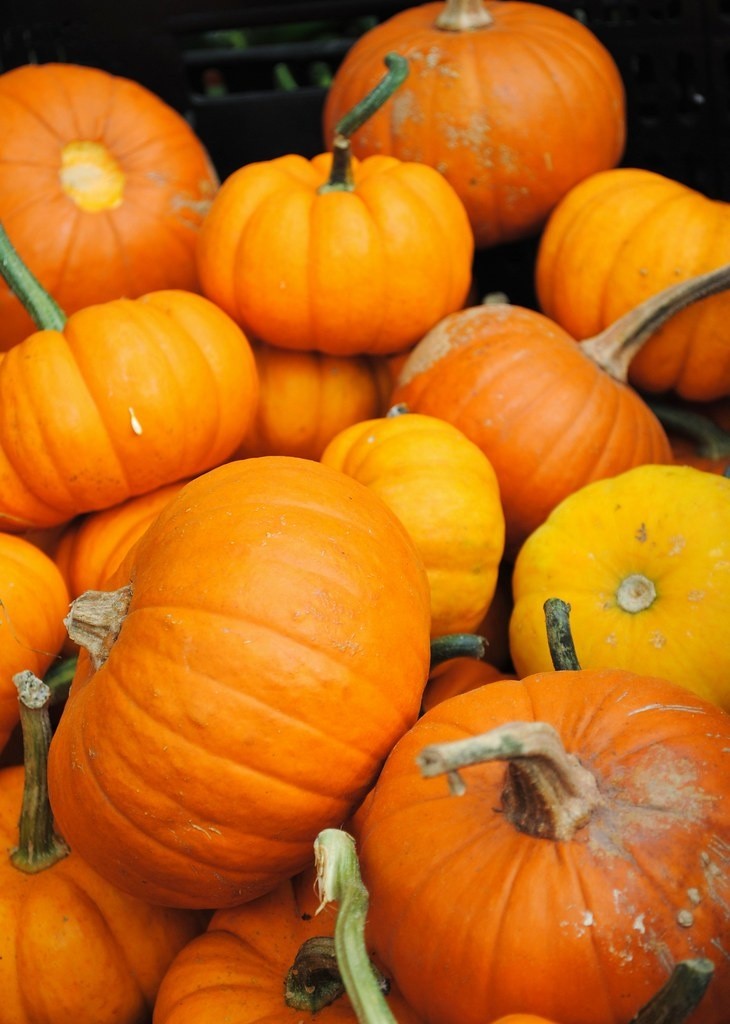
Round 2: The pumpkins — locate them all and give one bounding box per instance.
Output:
[0,1,730,1024]
